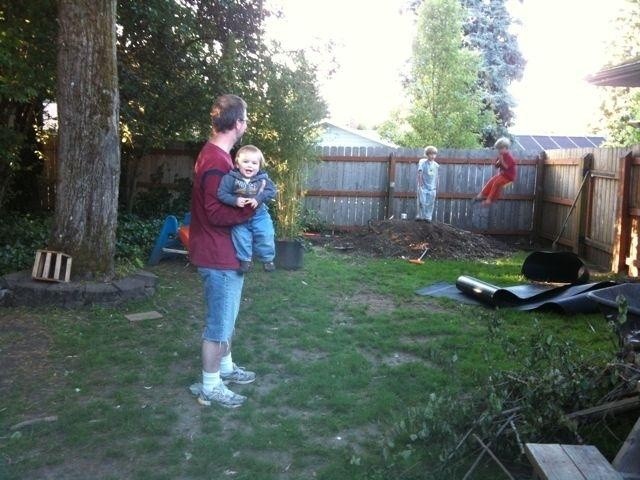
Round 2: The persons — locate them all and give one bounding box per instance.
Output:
[473,137,518,207]
[189,94,266,409]
[414,145,440,222]
[217,145,277,273]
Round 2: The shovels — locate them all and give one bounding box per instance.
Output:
[552,171,590,251]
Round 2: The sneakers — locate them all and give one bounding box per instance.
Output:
[198,378,248,408]
[264,261,275,272]
[239,259,254,272]
[220,362,257,385]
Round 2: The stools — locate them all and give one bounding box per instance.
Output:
[523,442,625,480]
